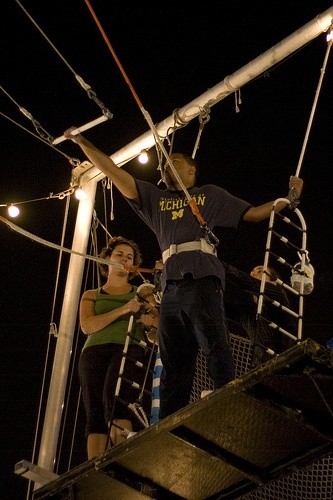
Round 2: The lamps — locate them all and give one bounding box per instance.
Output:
[138,150,148,164]
[8,206,20,218]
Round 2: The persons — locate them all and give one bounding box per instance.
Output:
[65,126,302,423]
[77,234,161,462]
